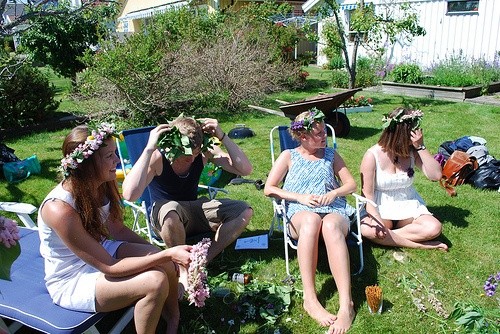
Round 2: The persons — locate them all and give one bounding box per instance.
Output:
[354,106,448,252]
[37,125,192,334]
[122,115,254,265]
[264,107,358,334]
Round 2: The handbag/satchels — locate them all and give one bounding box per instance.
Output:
[442,149,472,186]
[3,155,41,184]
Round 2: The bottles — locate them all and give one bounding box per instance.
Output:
[228,270,253,284]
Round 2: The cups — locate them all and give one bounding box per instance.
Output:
[262,263,276,282]
[367,299,383,317]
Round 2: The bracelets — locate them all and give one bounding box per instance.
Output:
[219,133,225,141]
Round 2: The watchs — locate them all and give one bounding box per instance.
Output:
[416,145,425,151]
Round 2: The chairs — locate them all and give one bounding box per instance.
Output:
[117,125,255,255]
[269,124,377,277]
[0,202,185,334]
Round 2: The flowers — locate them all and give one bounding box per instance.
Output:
[0,216,21,282]
[187,238,210,309]
[156,115,214,165]
[391,271,451,319]
[60,119,117,178]
[380,109,424,130]
[290,106,325,132]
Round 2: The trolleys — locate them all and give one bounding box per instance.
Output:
[247,87,364,138]
